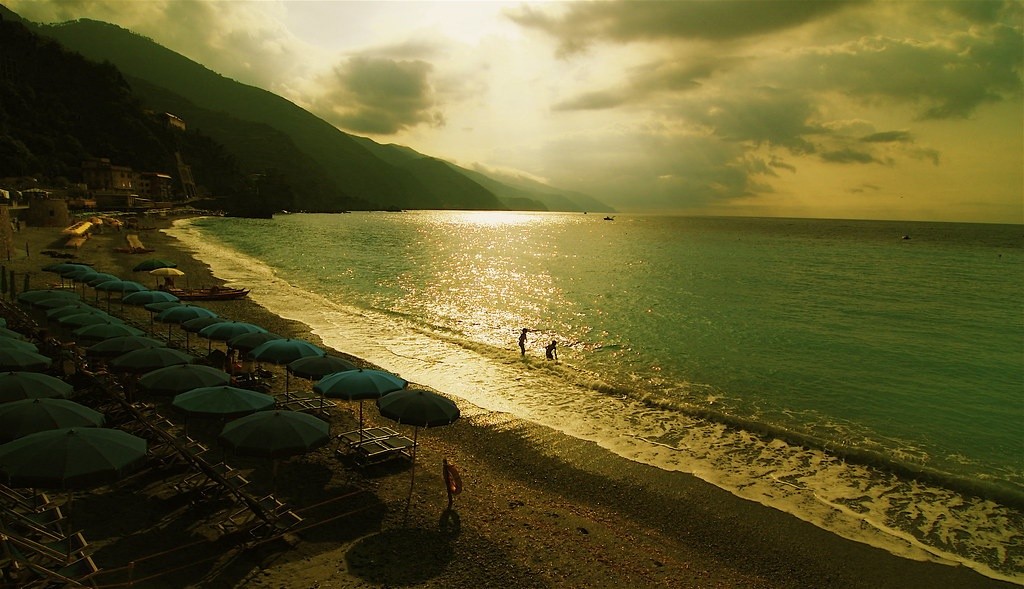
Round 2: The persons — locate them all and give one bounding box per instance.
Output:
[518,328,527,355]
[545,340,558,361]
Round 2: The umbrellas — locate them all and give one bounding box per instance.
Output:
[0,264,460,563]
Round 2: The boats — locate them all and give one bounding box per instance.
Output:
[166,286,251,299]
[604,216,614,220]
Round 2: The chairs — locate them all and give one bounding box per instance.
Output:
[0,290,420,589]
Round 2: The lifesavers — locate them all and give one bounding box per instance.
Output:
[448,464,462,495]
[439,508,461,538]
